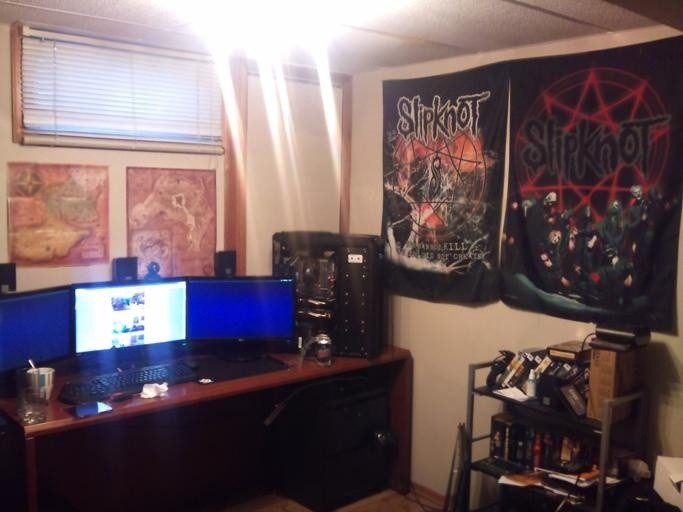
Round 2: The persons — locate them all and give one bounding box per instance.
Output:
[424,152,682,311]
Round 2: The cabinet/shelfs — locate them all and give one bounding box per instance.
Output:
[270,390,392,511]
[462,357,646,512]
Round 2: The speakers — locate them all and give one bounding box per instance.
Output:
[112,256,138,281]
[214,250,236,277]
[0,263,16,290]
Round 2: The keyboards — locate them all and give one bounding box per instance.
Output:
[58,358,198,407]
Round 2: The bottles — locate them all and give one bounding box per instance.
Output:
[526,368,535,398]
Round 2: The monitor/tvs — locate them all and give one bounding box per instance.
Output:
[0,284,73,376]
[188,275,296,363]
[71,275,188,371]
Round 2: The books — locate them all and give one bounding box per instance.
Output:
[488,340,625,498]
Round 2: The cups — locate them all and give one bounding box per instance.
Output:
[14,365,48,423]
[28,366,53,401]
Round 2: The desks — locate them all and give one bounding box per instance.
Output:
[1,333,411,511]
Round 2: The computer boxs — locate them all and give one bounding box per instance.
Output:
[272,230,386,361]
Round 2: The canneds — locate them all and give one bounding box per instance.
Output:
[317,335,332,366]
